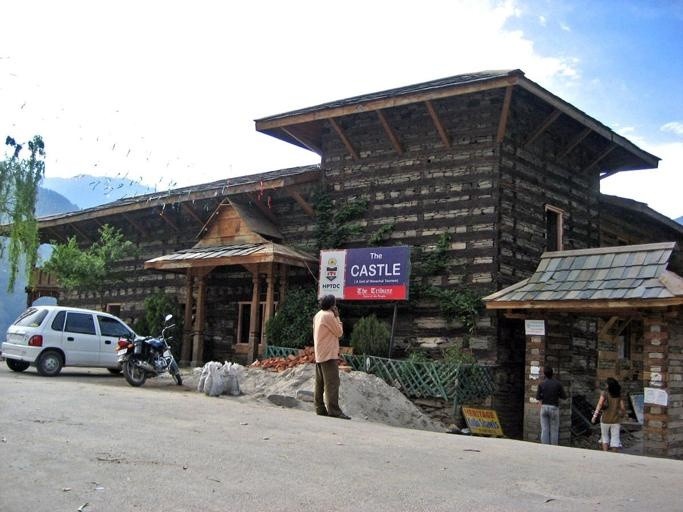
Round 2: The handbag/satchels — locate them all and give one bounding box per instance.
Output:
[601,399,608,410]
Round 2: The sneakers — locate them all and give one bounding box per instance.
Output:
[338,413,348,418]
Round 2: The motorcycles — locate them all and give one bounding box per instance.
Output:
[115,314,182,386]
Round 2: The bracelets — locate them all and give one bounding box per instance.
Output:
[593,411,599,417]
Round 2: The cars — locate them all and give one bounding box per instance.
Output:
[1,305,146,377]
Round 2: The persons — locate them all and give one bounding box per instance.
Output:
[591,377,627,453]
[536,367,567,445]
[312,295,351,419]
